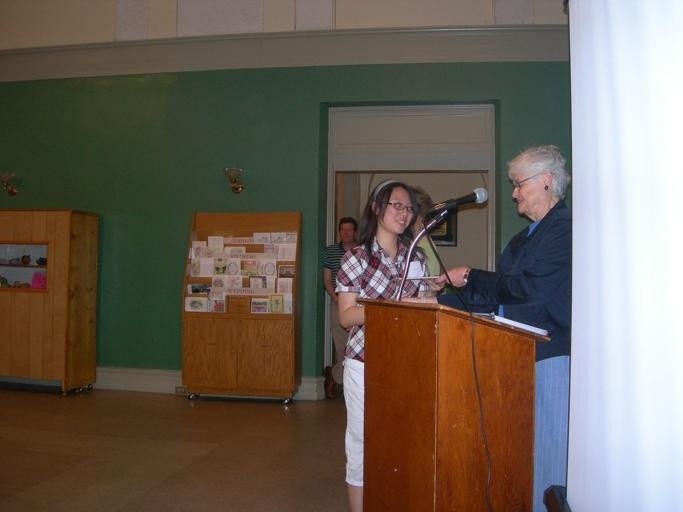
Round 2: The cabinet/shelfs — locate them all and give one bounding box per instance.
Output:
[181,209,304,405]
[0,243,47,293]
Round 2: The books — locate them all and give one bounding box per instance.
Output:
[184,230,297,314]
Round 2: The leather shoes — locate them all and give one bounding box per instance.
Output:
[324,366,337,401]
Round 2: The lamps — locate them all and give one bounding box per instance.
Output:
[222,166,245,194]
[0,172,18,197]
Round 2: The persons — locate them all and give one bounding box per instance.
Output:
[320,217,360,400]
[407,184,440,282]
[333,179,445,512]
[401,142,572,511]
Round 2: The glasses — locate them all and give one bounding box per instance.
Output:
[387,202,414,214]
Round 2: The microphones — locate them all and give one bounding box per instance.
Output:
[427,188,488,216]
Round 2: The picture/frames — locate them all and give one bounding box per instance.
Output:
[426,203,458,246]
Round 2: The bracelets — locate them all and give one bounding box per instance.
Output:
[421,296,426,303]
[463,269,470,286]
